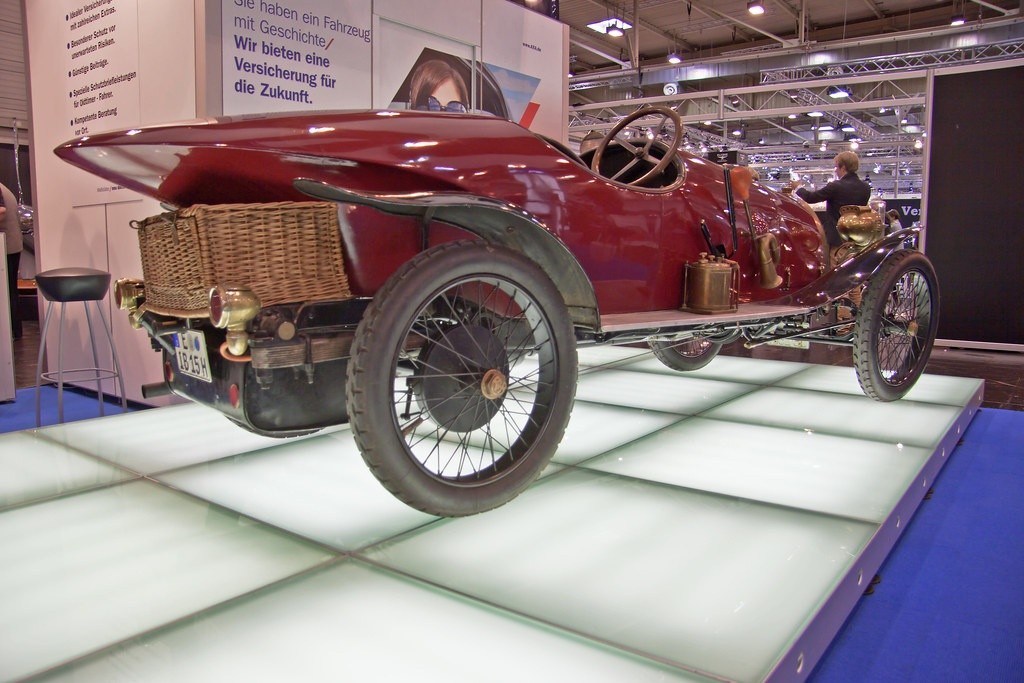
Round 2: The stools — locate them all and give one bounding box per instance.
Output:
[33,268,128,427]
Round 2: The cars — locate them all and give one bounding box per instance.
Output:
[52,108,943,519]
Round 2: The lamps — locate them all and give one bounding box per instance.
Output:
[667,36,683,64]
[747,0,765,15]
[827,86,852,98]
[606,1,626,38]
[950,15,966,26]
[841,124,856,132]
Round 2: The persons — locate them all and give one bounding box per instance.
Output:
[884,209,906,304]
[0,183,24,343]
[408,61,471,114]
[791,152,872,335]
